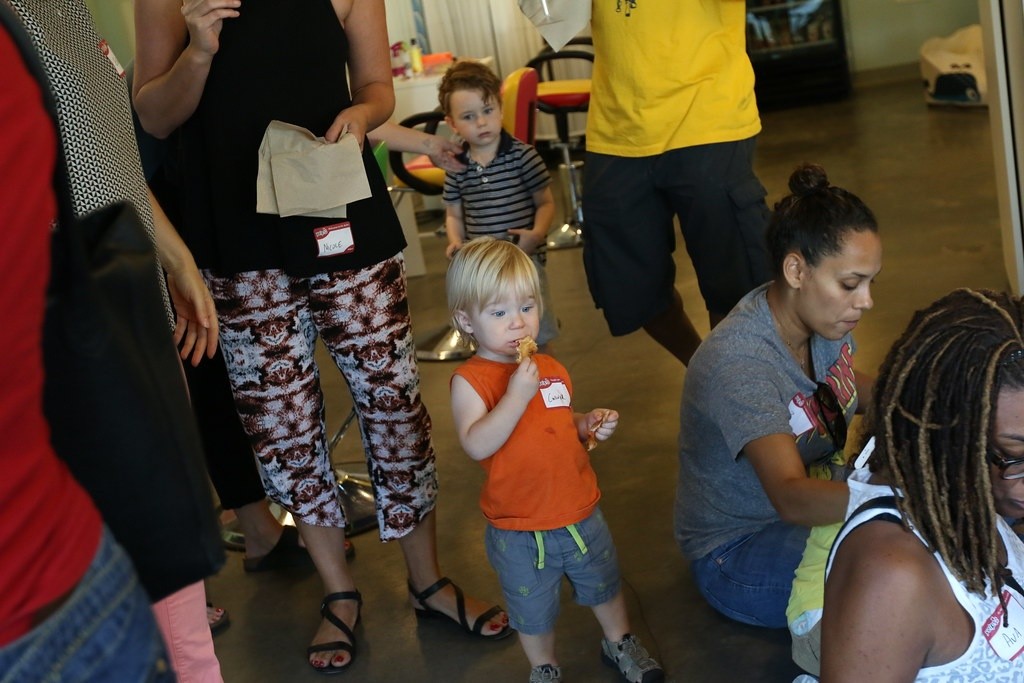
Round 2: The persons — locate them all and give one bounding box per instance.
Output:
[671,160,880,632]
[818,285,1024,683]
[437,60,561,357]
[131,0,519,672]
[445,234,668,683]
[1,0,222,683]
[580,1,785,366]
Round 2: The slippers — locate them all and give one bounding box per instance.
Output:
[243,525,356,572]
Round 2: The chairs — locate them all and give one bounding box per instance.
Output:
[524,36,595,250]
[385,65,539,361]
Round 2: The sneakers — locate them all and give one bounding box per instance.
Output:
[529,664,563,683]
[601,633,666,683]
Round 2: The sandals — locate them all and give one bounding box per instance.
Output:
[306,577,516,674]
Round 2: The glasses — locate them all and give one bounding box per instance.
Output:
[987,442,1024,481]
[814,381,846,453]
